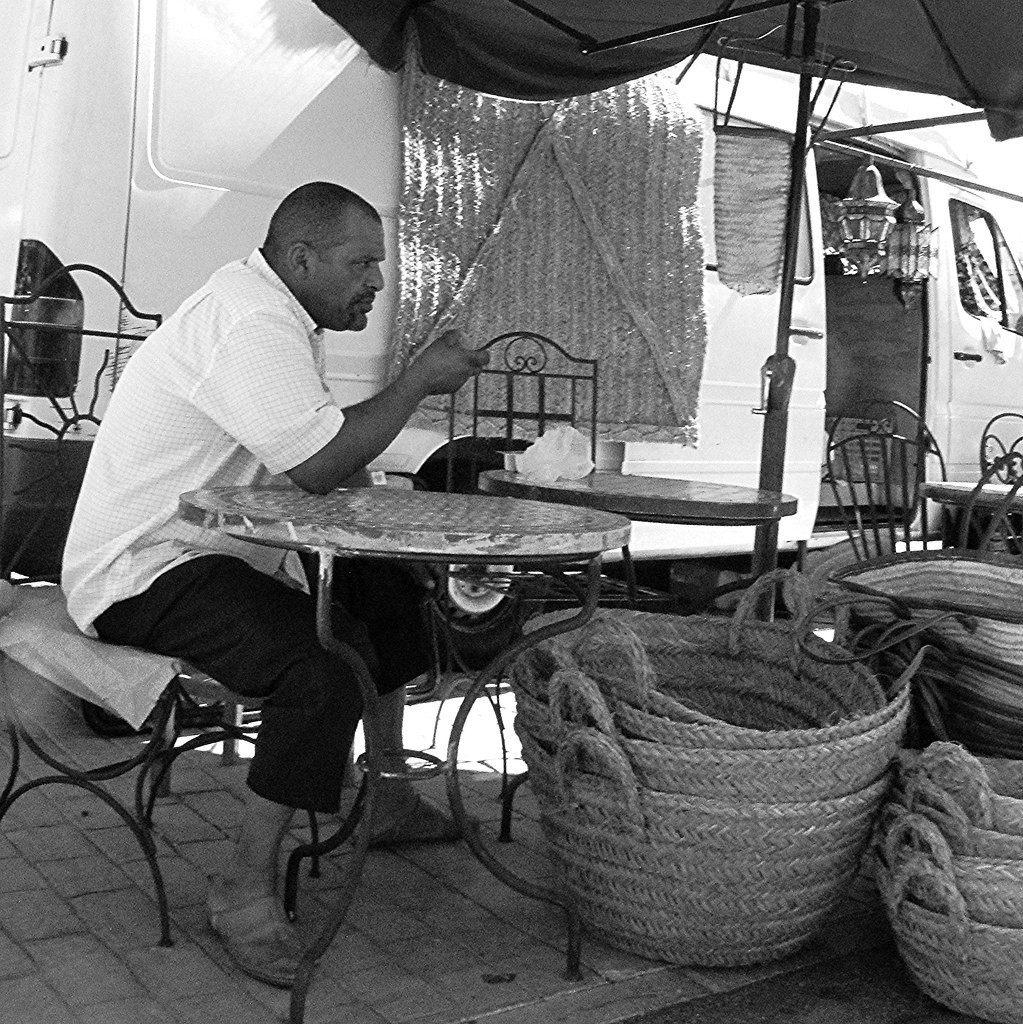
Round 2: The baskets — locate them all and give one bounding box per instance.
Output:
[510,546,1023,1024]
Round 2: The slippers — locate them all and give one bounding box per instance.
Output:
[207,894,303,986]
[349,794,453,846]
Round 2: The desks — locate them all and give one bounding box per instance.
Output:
[476,467,800,843]
[177,482,631,1024]
[918,482,1023,561]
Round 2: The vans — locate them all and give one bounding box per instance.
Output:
[0,1,1023,672]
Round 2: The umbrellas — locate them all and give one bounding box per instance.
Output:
[311,0,1023,621]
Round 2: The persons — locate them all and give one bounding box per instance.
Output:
[61,183,490,987]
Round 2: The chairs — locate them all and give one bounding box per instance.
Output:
[826,397,949,563]
[448,330,667,841]
[0,264,319,949]
[956,451,1023,561]
[980,413,1023,555]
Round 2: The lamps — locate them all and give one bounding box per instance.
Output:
[838,159,902,279]
[888,188,933,310]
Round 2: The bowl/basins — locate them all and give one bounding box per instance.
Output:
[497,451,526,471]
